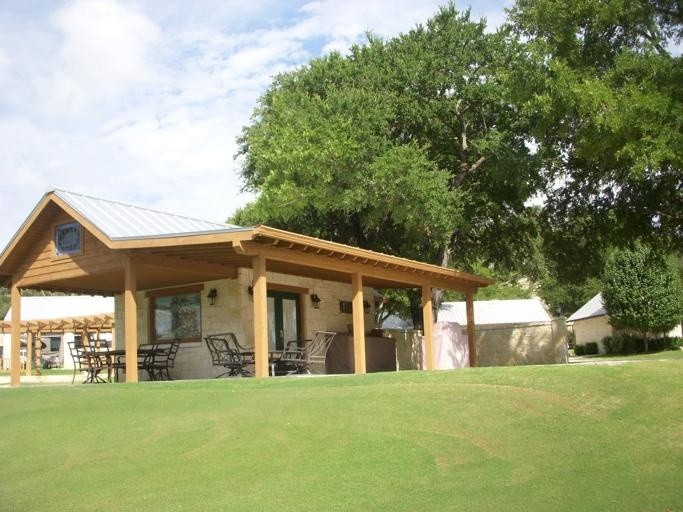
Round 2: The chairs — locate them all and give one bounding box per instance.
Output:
[67,338,182,384]
[203,331,337,379]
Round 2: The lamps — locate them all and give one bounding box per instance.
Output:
[206,288,217,305]
[364,301,371,313]
[311,295,321,308]
